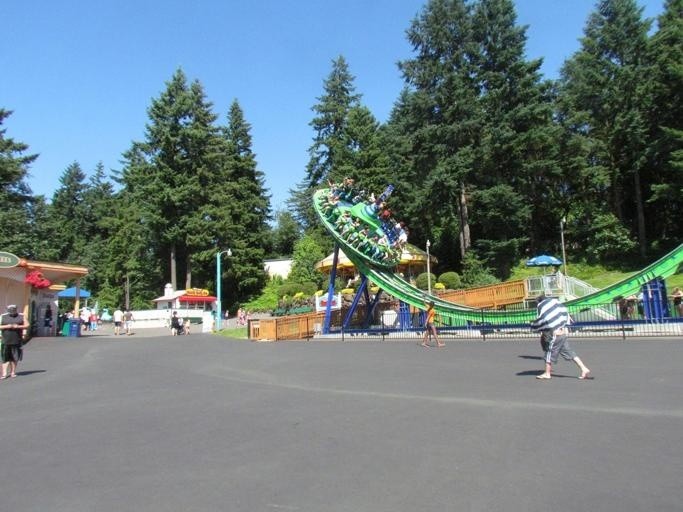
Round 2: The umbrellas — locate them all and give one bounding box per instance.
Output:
[525,255,563,275]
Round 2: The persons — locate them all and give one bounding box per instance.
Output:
[318,175,410,272]
[122,309,136,335]
[236,307,252,325]
[206,303,212,311]
[224,310,229,320]
[530,294,591,380]
[58,302,101,332]
[619,288,647,321]
[170,311,191,337]
[113,306,124,336]
[44,304,52,327]
[421,294,445,348]
[0,305,31,380]
[671,287,683,318]
[552,265,560,275]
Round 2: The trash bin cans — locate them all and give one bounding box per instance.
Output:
[62,318,81,337]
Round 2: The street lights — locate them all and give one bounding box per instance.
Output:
[216,248,232,331]
[426,239,431,294]
[560,216,567,276]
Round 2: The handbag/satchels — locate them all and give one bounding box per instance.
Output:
[540,336,549,352]
